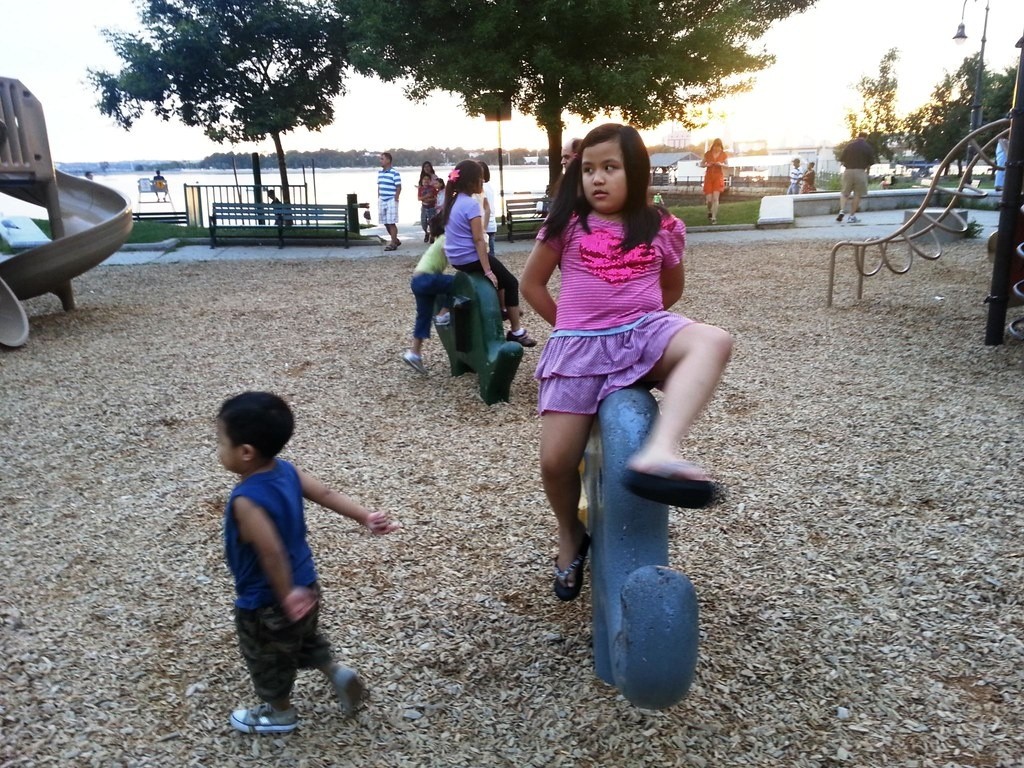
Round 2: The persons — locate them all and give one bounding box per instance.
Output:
[267,190,283,224]
[376,153,401,251]
[518,123,732,603]
[401,160,536,374]
[559,138,583,171]
[835,131,874,224]
[993,111,1009,190]
[214,392,401,734]
[153,169,168,202]
[85,171,92,180]
[786,158,815,194]
[699,138,728,222]
[652,191,664,205]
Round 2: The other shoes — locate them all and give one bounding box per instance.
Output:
[395,243,401,246]
[384,244,396,251]
[430,239,434,243]
[425,233,429,242]
[708,213,717,225]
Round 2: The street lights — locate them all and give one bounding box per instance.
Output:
[951,0,989,186]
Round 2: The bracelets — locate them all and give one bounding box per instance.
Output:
[485,271,492,276]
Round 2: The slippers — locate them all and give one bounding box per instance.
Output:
[619,466,716,511]
[552,532,591,601]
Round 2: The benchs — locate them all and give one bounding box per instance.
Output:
[505,197,551,242]
[206,202,350,250]
[135,177,178,223]
[903,208,969,243]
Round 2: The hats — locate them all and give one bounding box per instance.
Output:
[792,158,800,163]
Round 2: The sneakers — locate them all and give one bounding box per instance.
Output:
[501,306,524,322]
[506,328,537,347]
[229,701,300,733]
[404,347,428,375]
[847,216,862,223]
[432,311,450,326]
[332,667,363,714]
[837,210,845,221]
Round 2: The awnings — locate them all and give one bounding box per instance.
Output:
[650,152,702,184]
